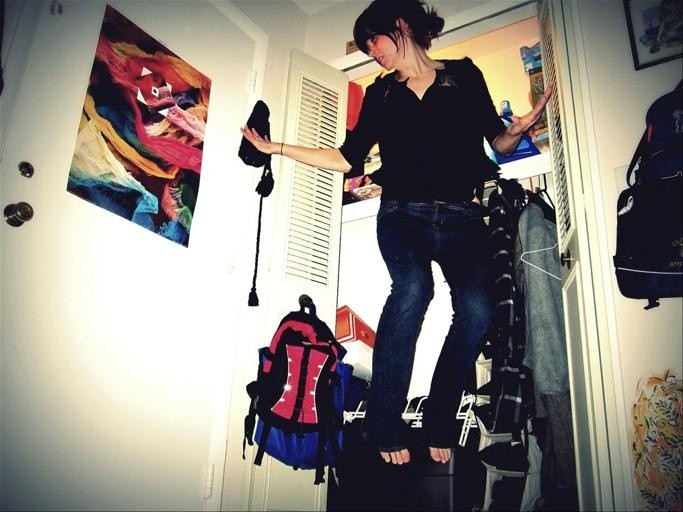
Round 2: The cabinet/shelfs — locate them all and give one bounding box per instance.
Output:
[323,1,584,512]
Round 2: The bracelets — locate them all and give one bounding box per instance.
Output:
[280,142,283,154]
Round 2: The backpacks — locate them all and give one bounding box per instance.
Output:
[613,72,682,310]
[242,295,353,484]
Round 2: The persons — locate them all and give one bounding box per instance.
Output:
[242,0,554,464]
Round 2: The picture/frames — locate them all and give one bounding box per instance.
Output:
[623,1,682,73]
